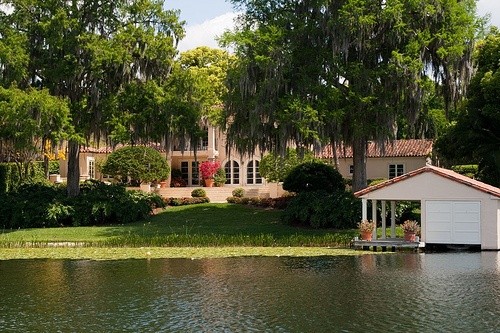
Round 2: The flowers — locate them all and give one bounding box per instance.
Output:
[357,220,375,233]
[400,220,419,232]
[201,161,220,179]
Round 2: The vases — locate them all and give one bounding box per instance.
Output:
[160,182,166,188]
[405,232,416,241]
[205,179,214,187]
[361,232,372,240]
